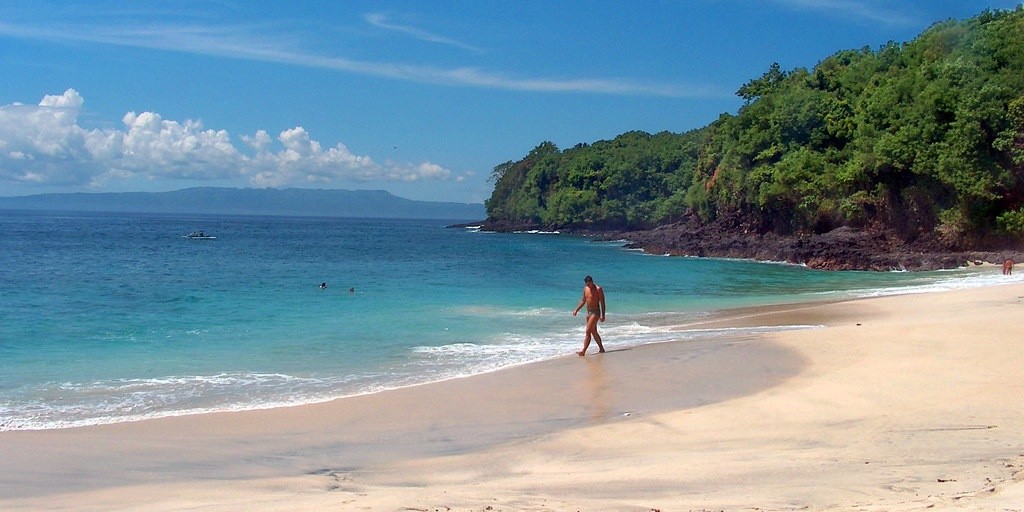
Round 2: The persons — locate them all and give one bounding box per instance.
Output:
[1001,258,1013,275]
[572,275,606,356]
[193,230,210,237]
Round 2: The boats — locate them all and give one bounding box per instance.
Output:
[181,236,217,240]
